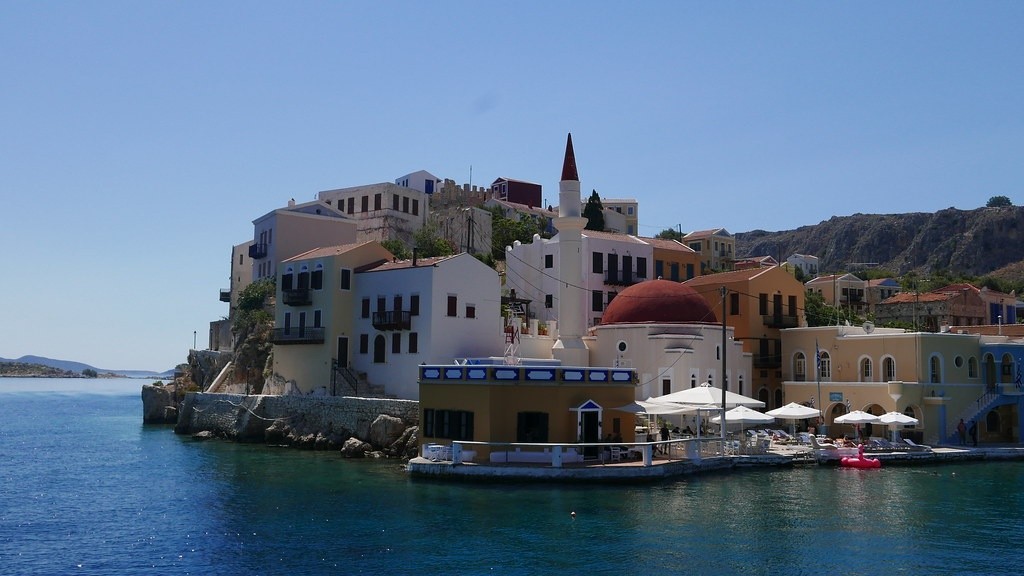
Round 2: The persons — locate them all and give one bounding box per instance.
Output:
[604,433,628,459]
[646,424,704,458]
[957,419,967,446]
[969,421,978,446]
[843,423,872,448]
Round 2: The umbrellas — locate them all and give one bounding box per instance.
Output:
[634,382,776,451]
[765,402,822,439]
[869,412,918,441]
[834,410,880,439]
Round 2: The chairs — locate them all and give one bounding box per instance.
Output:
[603,429,931,456]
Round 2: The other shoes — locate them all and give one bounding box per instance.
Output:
[964,444,966,445]
[664,452,668,455]
[959,444,962,445]
[973,444,977,447]
[652,455,656,458]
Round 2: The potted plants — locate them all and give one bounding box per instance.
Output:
[538,322,547,335]
